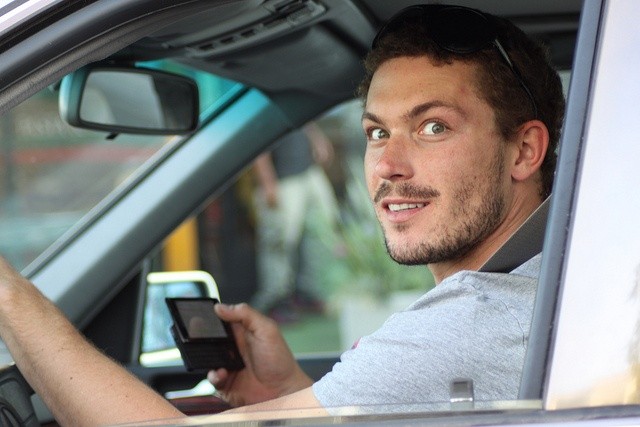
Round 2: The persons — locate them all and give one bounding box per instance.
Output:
[244,121,353,313]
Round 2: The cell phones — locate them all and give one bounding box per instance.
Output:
[164,296,246,377]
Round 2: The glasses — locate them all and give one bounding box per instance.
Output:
[371,5,537,116]
